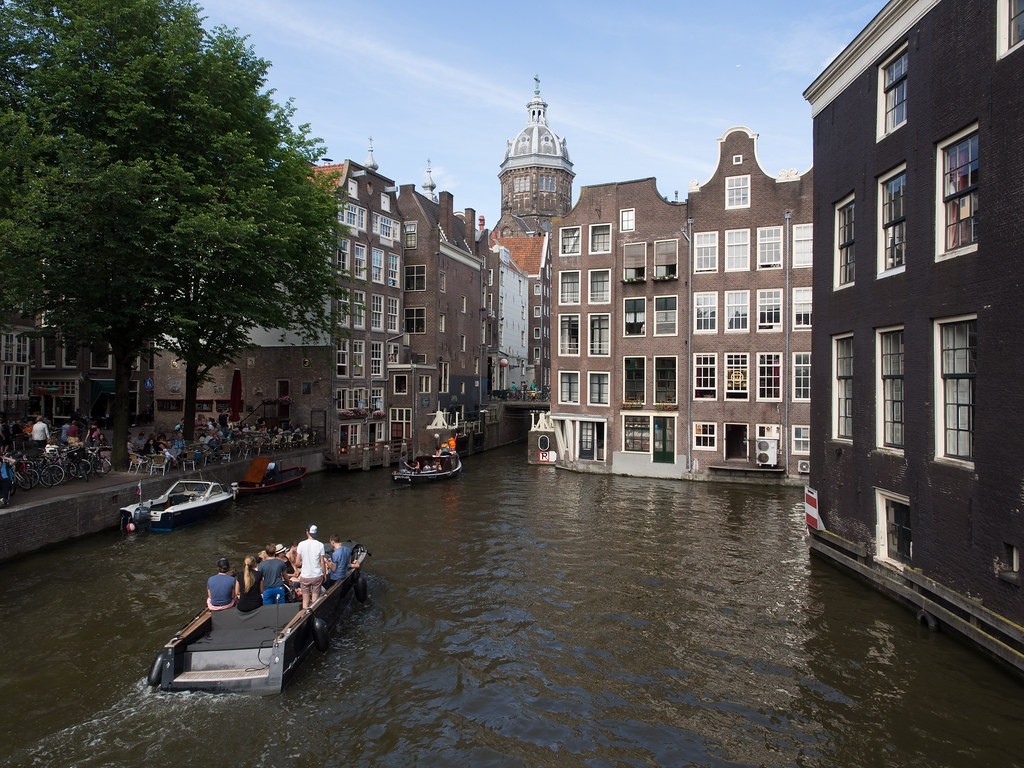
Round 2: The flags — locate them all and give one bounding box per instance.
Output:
[136,482,141,496]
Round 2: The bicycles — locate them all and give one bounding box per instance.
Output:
[0,443,112,508]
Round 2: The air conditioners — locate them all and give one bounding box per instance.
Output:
[798,460,810,472]
[403,335,411,346]
[756,440,777,464]
[388,342,399,354]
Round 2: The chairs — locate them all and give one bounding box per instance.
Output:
[128,414,321,476]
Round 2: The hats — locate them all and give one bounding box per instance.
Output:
[308,524,318,538]
[275,544,286,554]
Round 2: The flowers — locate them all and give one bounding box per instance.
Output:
[373,410,385,417]
[338,408,366,416]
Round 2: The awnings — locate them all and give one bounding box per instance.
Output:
[90,381,115,409]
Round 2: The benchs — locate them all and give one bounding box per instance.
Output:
[186,602,301,651]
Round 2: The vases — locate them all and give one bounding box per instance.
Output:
[373,415,381,420]
[339,415,351,420]
[354,415,364,419]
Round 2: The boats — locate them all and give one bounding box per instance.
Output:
[392,450,462,483]
[119,479,233,535]
[148,541,371,695]
[238,457,307,494]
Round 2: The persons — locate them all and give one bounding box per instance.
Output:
[399,433,459,474]
[510,379,538,401]
[206,523,362,614]
[0,407,316,508]
